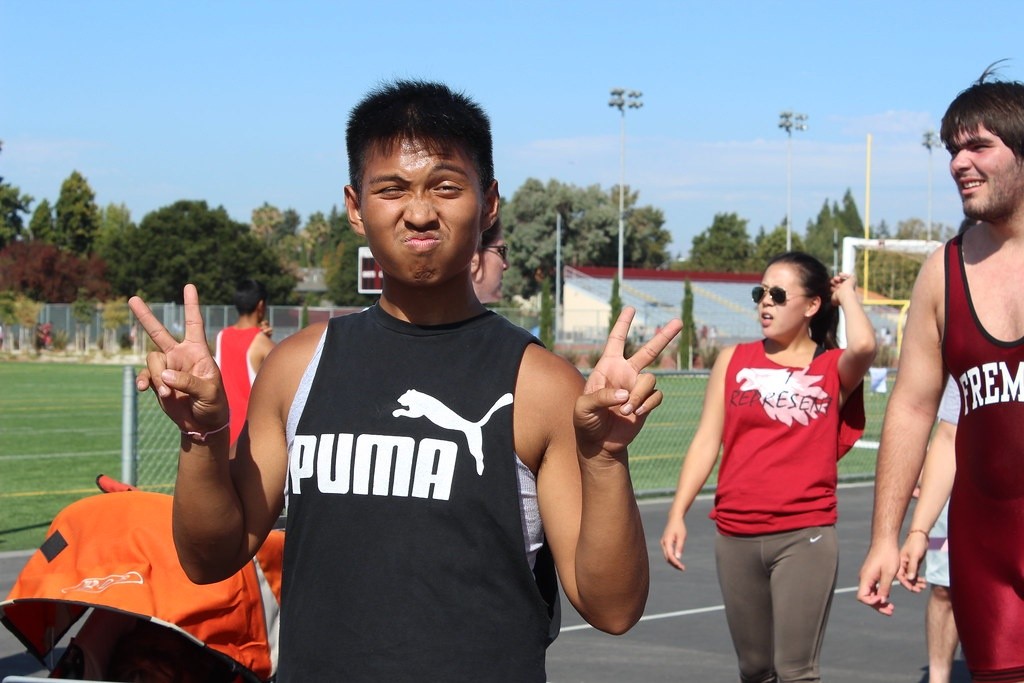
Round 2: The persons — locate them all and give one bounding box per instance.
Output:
[896,364,964,681]
[205,277,281,461]
[33,321,52,359]
[658,251,878,681]
[454,205,511,304]
[858,78,1023,682]
[123,80,683,683]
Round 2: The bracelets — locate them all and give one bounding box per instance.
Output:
[903,527,931,545]
[178,422,231,442]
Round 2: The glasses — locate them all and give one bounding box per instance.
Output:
[482,243,509,259]
[752,285,809,305]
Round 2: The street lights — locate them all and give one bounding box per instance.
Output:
[922,129,943,241]
[607,83,646,301]
[778,111,811,254]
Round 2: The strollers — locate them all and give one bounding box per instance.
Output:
[1,474,285,683]
